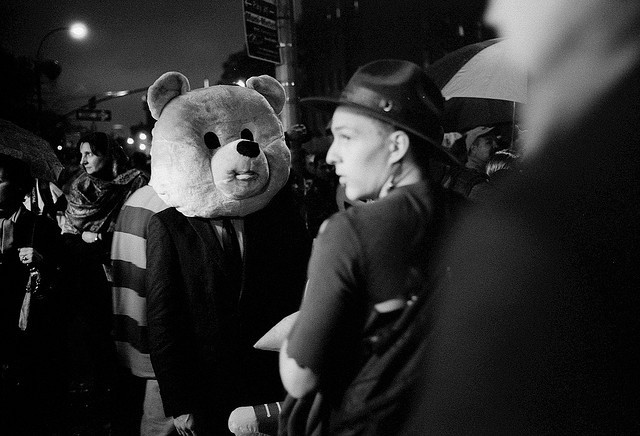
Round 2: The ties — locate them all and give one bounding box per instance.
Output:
[222,218,241,299]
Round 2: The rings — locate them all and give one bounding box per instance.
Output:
[22,256,26,261]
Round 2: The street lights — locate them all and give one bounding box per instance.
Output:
[34,23,87,107]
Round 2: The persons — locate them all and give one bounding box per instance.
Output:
[311,155,336,201]
[305,154,315,194]
[457,125,497,198]
[63,132,149,242]
[111,186,173,436]
[0,155,61,269]
[483,151,515,180]
[146,70,300,436]
[276,55,459,435]
[431,1,637,432]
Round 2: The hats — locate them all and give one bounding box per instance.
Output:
[465,125,493,152]
[301,58,460,163]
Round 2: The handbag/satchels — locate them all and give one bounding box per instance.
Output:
[22,211,85,323]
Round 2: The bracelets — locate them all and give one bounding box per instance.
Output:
[95,233,98,241]
